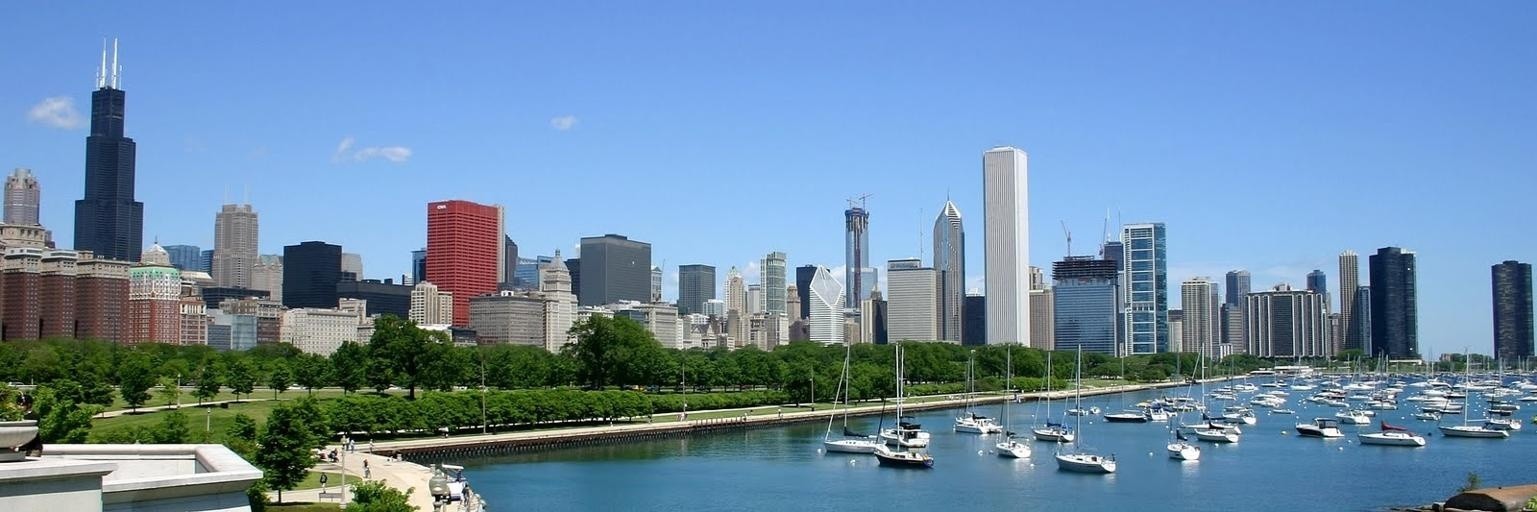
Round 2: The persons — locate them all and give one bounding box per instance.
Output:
[328,447,339,460]
[340,434,346,447]
[345,436,350,450]
[319,471,327,493]
[350,438,356,453]
[455,469,461,481]
[461,484,470,505]
[362,458,368,467]
[319,449,328,459]
[368,438,375,454]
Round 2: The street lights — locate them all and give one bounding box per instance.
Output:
[176,372,181,409]
[206,407,210,431]
[338,435,348,511]
[428,467,449,512]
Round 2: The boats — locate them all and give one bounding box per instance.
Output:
[1295,417,1347,439]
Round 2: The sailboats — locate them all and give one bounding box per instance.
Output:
[1051,344,1118,474]
[1410,346,1537,430]
[1166,343,1201,462]
[1143,341,1292,443]
[1437,347,1511,439]
[878,342,931,447]
[1291,353,1315,391]
[952,353,1005,434]
[1102,348,1149,423]
[822,341,886,455]
[995,344,1033,458]
[1354,350,1426,446]
[872,344,935,467]
[1310,350,1408,425]
[1031,351,1077,444]
